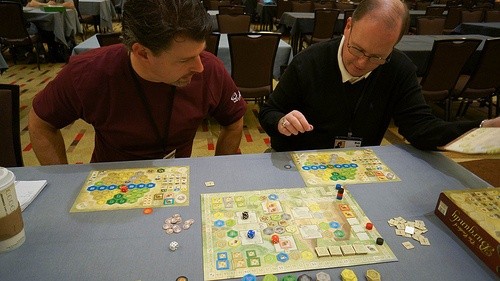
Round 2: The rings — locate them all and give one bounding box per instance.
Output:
[283,120,288,128]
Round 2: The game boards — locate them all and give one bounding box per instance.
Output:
[199,183,400,281]
[287,148,402,188]
[68,164,191,214]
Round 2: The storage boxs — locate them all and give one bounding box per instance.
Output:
[40,5,66,12]
[434,188,500,278]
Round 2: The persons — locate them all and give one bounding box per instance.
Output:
[257,0,500,152]
[27,0,247,170]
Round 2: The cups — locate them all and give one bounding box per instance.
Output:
[0,166,27,251]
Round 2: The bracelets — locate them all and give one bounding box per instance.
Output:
[480,119,488,127]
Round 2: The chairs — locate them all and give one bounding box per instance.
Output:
[0,0,500,167]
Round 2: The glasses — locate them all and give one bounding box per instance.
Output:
[346,22,393,65]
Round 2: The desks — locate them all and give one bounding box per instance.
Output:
[452,21,500,38]
[396,33,494,78]
[77,0,120,33]
[71,33,293,81]
[22,6,84,64]
[0,143,500,281]
[408,10,449,28]
[277,12,345,56]
[206,9,219,31]
